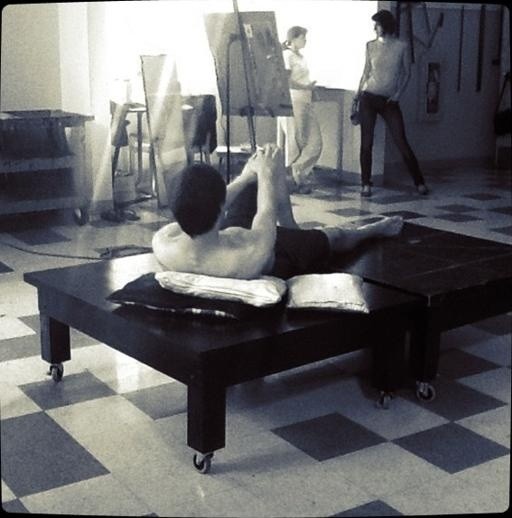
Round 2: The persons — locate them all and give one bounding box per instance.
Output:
[279,25,324,195]
[350,8,431,197]
[150,142,405,281]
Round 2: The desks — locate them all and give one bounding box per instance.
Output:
[22,250,426,475]
[317,217,512,403]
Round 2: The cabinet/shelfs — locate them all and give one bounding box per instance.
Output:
[0,108,95,226]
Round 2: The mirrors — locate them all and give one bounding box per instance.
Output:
[140,54,190,209]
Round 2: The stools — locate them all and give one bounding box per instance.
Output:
[215,146,252,174]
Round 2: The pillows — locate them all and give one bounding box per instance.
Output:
[105,270,370,320]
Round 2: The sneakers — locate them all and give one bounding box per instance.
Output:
[416,184,428,195]
[286,166,311,194]
[360,184,371,197]
